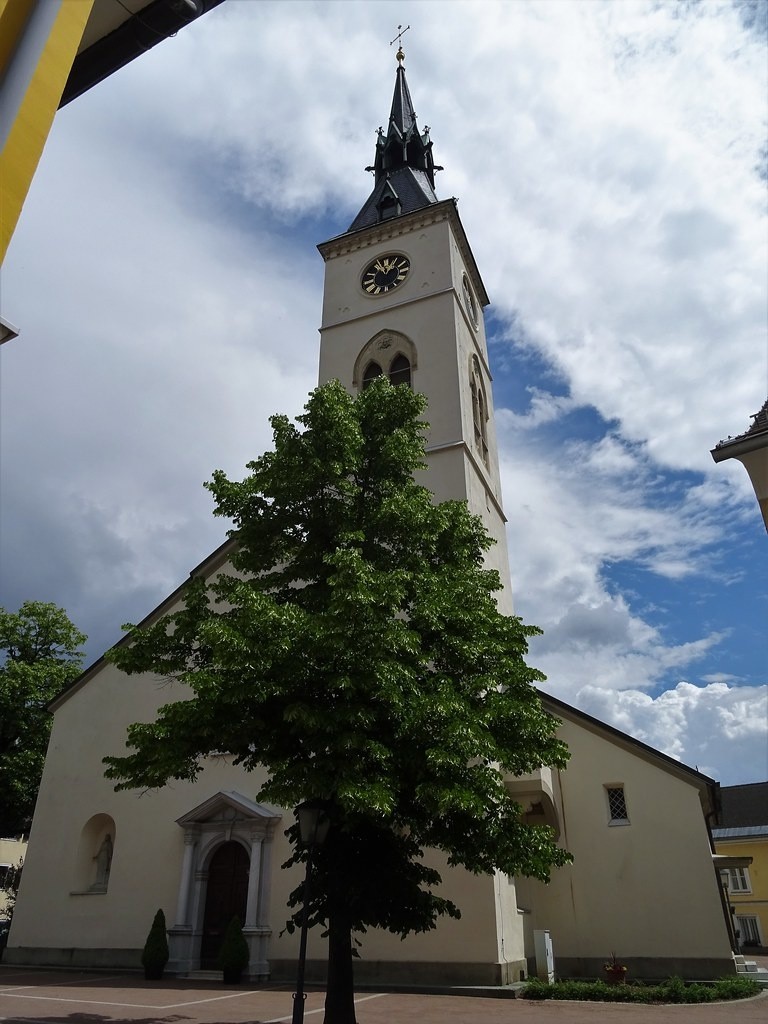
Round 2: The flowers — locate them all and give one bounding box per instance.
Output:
[601,952,631,974]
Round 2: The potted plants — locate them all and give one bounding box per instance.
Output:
[221,915,250,985]
[741,939,763,955]
[140,909,169,981]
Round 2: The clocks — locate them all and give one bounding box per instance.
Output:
[360,253,410,295]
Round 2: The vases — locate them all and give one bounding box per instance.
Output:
[607,971,625,985]
